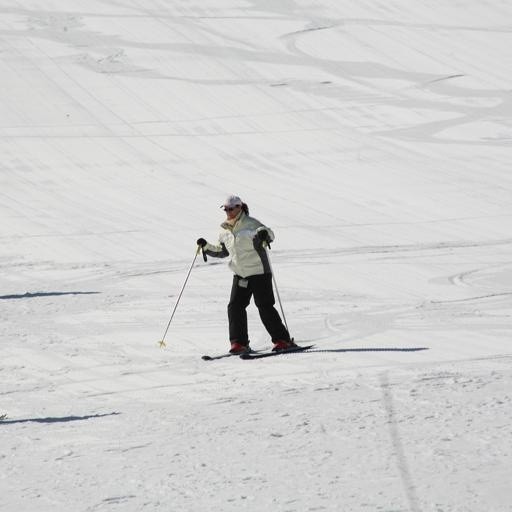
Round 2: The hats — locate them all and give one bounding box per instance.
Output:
[220,196,242,208]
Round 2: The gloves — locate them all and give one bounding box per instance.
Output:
[257,230,268,241]
[197,238,207,247]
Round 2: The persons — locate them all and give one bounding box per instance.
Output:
[195,195,298,354]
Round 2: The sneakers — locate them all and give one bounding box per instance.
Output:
[272,340,296,351]
[229,343,250,353]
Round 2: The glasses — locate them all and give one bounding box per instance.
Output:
[224,207,237,211]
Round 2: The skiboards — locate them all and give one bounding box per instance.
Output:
[201,344,313,361]
